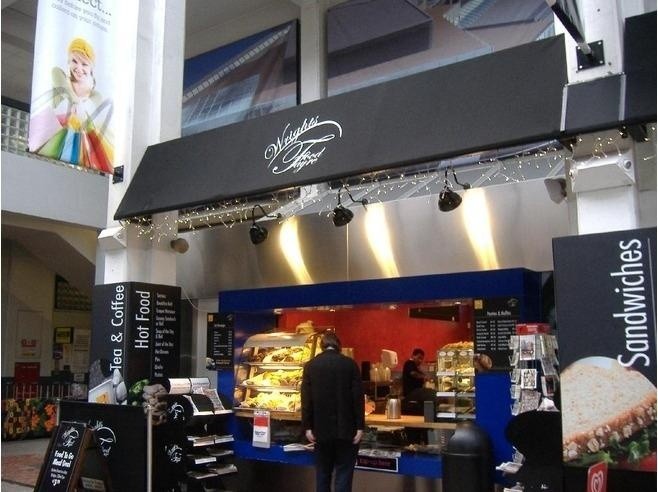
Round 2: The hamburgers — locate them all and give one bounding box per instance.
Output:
[559,356,657,472]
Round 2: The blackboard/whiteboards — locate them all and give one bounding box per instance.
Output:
[34,422,87,492]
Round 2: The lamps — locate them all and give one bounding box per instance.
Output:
[438,166,471,212]
[333,185,369,227]
[249,205,281,245]
[545,178,567,204]
[171,237,189,254]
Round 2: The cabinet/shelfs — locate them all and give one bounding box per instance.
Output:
[158,394,238,492]
[435,348,475,420]
[233,325,336,420]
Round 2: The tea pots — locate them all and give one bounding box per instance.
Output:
[385,393,402,419]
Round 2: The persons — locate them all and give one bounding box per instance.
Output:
[300,333,366,492]
[33,36,117,175]
[401,349,432,394]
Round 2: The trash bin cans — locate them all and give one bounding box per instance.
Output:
[441,421,493,492]
